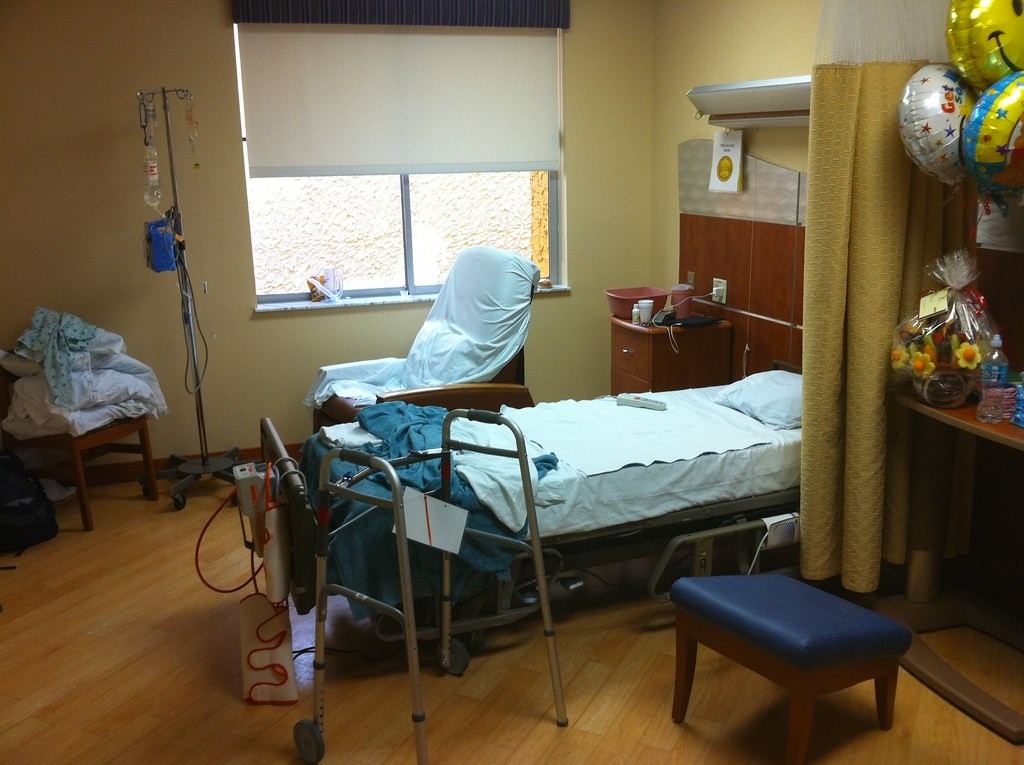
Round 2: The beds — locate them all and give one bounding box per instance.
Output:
[258,357,811,650]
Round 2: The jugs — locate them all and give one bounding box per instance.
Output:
[306,267,343,303]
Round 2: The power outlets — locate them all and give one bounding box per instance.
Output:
[712,278,728,304]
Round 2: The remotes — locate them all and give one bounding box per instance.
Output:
[617,393,667,412]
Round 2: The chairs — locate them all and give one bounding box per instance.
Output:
[303,248,540,438]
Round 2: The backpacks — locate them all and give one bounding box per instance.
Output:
[0,451,59,556]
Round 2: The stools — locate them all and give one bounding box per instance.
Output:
[3,412,158,529]
[671,575,914,764]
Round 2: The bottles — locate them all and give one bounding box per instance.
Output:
[638,299,654,327]
[975,333,1008,424]
[631,303,640,325]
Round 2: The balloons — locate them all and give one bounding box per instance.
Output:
[899,0,1024,197]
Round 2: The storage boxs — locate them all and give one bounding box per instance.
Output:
[606,284,672,317]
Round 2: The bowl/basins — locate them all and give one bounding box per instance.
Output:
[604,286,670,319]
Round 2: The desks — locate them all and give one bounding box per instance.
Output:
[876,384,1024,744]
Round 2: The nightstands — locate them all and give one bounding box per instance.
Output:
[609,315,734,395]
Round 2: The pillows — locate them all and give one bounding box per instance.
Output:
[712,370,802,430]
